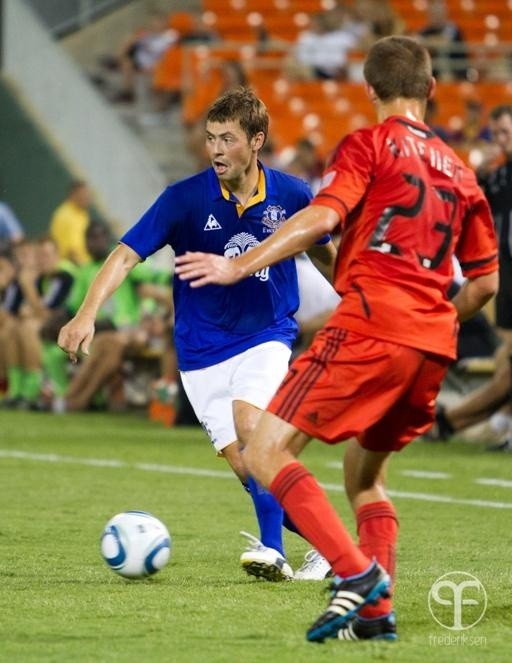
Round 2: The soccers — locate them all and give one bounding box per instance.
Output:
[101,510,171,581]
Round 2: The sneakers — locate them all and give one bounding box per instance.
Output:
[237,530,295,581]
[306,555,391,639]
[1,382,153,413]
[330,609,398,642]
[294,547,331,580]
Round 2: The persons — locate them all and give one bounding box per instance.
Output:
[0,0,511,644]
